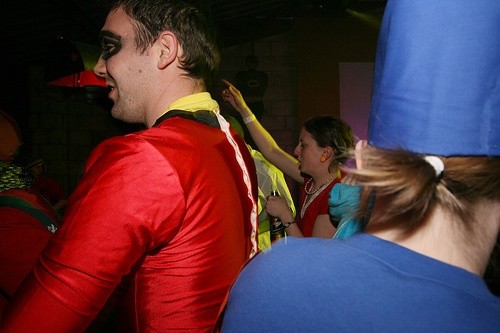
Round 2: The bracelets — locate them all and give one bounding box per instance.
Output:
[283,220,296,230]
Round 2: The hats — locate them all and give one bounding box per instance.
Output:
[367,0,500,156]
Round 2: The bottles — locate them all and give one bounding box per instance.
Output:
[269,184,286,246]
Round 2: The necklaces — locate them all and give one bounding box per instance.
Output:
[304,172,337,194]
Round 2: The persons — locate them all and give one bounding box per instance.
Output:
[0,0,258,333]
[222,114,297,253]
[221,75,356,242]
[222,0,500,332]
[0,106,69,316]
